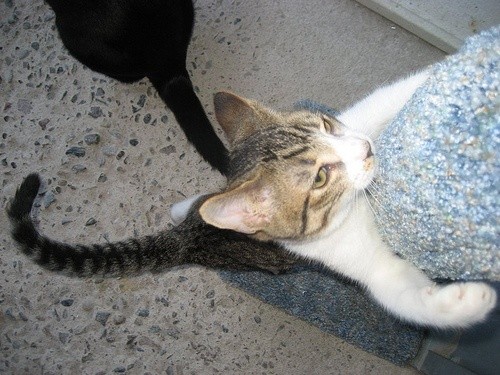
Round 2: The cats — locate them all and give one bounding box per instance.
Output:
[8,62,497,332]
